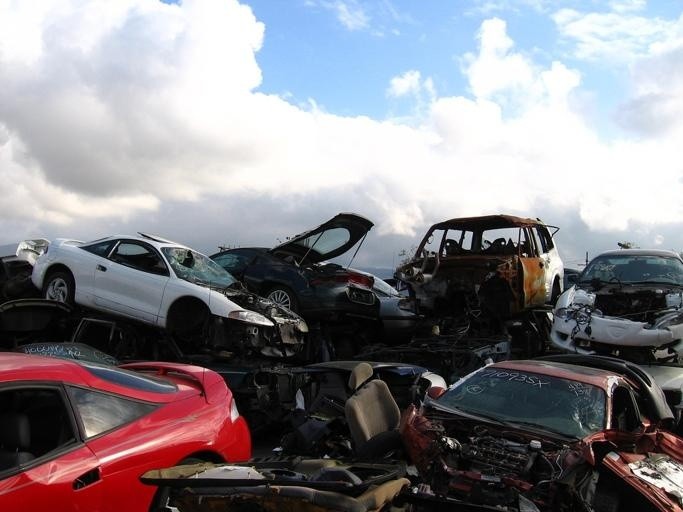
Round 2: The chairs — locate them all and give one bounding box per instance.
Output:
[344,363,400,461]
[0,410,35,472]
